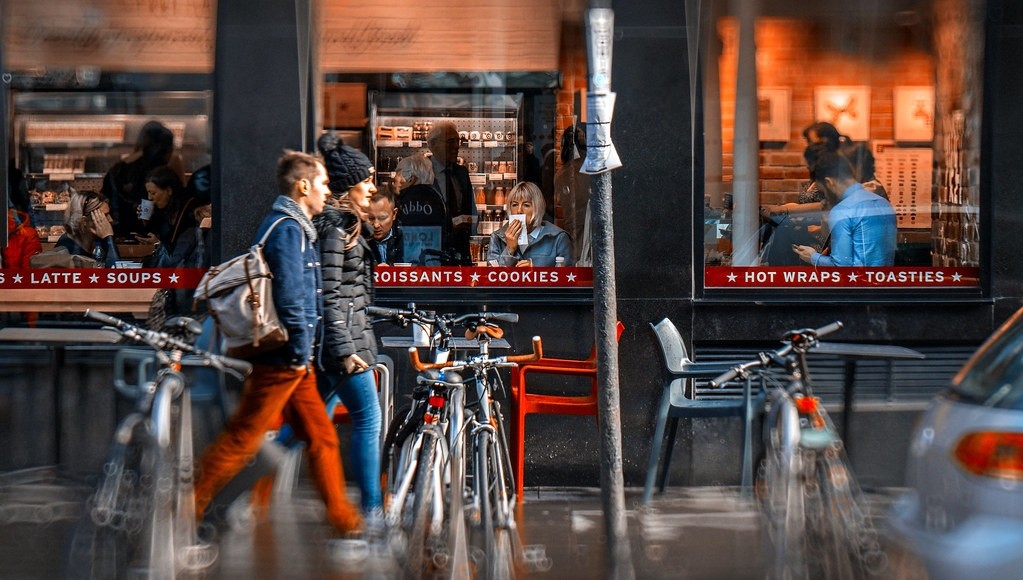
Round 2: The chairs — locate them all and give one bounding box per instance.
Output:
[636,317,762,502]
[509,318,628,501]
[240,354,396,525]
[112,316,230,464]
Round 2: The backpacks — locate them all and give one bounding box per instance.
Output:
[193,216,306,358]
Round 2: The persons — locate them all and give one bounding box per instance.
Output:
[394,153,452,266]
[193,152,366,540]
[193,132,386,535]
[368,185,404,267]
[840,141,889,200]
[53,189,136,323]
[792,151,897,267]
[102,122,183,244]
[135,165,196,312]
[487,181,577,267]
[759,141,838,217]
[426,119,480,266]
[801,121,853,148]
[187,163,212,268]
[6,207,42,328]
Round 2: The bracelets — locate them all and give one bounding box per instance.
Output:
[783,205,790,215]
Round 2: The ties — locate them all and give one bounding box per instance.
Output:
[443,169,458,218]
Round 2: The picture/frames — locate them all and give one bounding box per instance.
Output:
[811,84,871,142]
[755,85,794,142]
[891,85,935,141]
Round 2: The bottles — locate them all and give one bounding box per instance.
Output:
[377,155,515,262]
[555,257,564,267]
[106,235,119,268]
[93,242,106,268]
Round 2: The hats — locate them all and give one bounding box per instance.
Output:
[318,132,375,194]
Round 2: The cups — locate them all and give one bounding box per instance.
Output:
[115,260,143,268]
[139,199,155,220]
[394,263,414,267]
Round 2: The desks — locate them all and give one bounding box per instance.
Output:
[780,335,928,484]
[1,327,123,488]
[378,337,511,368]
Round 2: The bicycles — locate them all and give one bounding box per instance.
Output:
[80,305,256,580]
[707,318,881,580]
[361,303,544,580]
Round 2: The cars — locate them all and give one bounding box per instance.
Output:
[880,302,1023,579]
